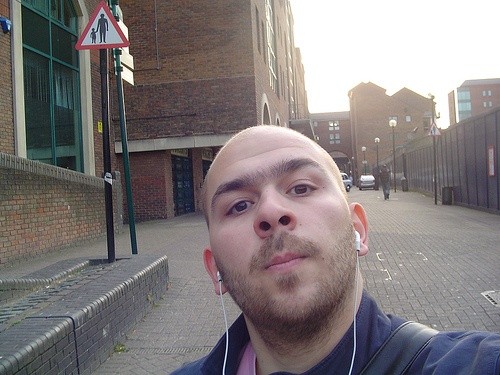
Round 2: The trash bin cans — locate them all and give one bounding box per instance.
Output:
[402,180,408,192]
[442,187,453,205]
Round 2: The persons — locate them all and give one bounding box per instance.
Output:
[169,123,500,375]
[379,164,391,200]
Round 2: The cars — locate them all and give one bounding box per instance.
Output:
[340,172,353,192]
[358,175,376,190]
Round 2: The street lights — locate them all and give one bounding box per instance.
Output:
[428,91,438,205]
[388,119,397,193]
[375,137,381,191]
[362,146,367,175]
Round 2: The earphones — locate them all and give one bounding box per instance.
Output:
[217,272,223,282]
[355,230,361,252]
